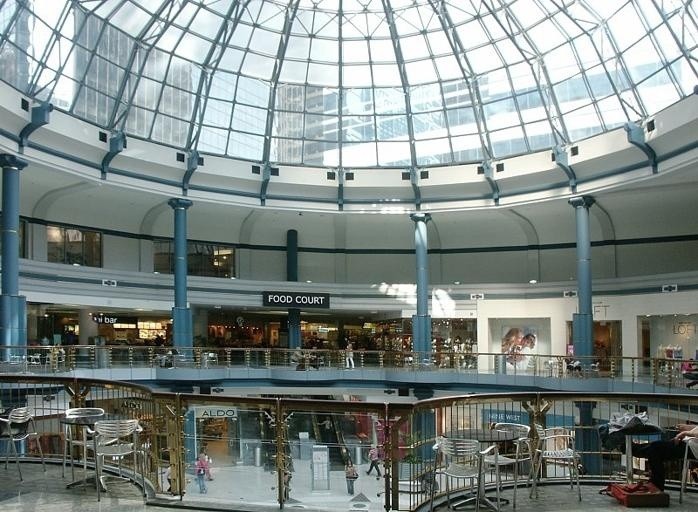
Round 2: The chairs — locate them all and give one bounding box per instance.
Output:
[290,355,325,370]
[8,352,66,373]
[153,352,219,370]
[680,436,698,504]
[659,365,693,389]
[0,406,145,503]
[543,359,600,379]
[437,422,582,512]
[403,356,435,371]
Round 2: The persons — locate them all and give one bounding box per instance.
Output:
[345,340,354,369]
[195,449,214,494]
[507,334,535,370]
[345,458,357,496]
[605,424,698,492]
[365,443,382,477]
[502,328,524,354]
[317,417,332,443]
[166,466,171,492]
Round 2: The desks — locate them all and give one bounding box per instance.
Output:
[599,431,662,495]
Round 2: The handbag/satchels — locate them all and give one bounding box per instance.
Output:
[598,478,670,508]
